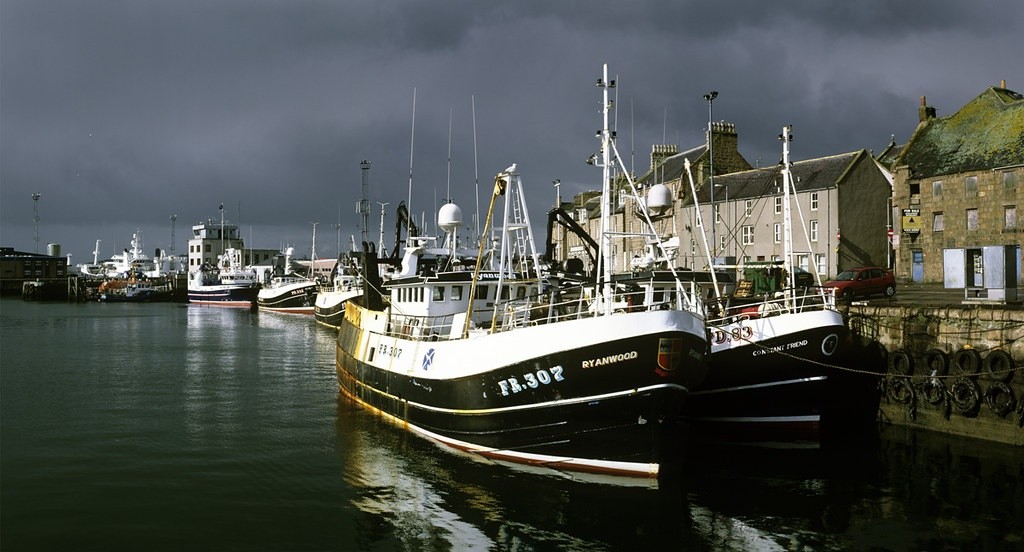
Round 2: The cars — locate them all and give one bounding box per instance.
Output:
[816,266,897,303]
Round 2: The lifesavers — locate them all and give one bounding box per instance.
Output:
[952,344,983,379]
[983,382,1016,416]
[889,348,914,376]
[921,348,950,378]
[984,348,1015,382]
[889,377,914,403]
[922,379,945,404]
[950,378,981,414]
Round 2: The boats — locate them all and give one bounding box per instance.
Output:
[332,61,712,479]
[124,287,158,302]
[186,248,257,311]
[314,235,369,326]
[257,248,314,313]
[700,124,858,456]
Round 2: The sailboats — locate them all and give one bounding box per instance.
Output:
[301,223,333,291]
